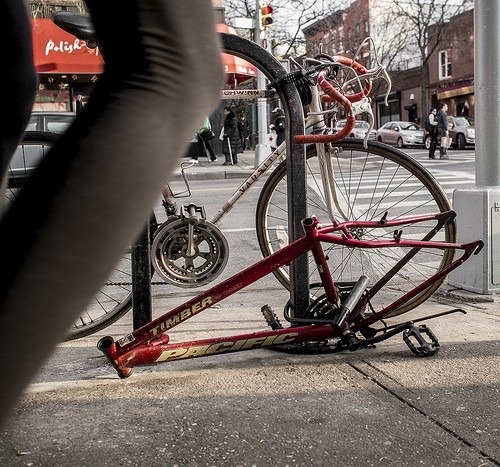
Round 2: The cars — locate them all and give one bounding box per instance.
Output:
[375,121,425,148]
[3,112,77,189]
[425,116,475,150]
[328,120,376,140]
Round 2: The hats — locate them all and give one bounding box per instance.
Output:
[272,108,281,112]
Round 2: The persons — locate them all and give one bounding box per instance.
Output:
[195,107,292,166]
[427,103,449,160]
[2,0,225,466]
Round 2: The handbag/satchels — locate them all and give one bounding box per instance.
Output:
[441,136,452,148]
[425,119,434,132]
[206,130,215,140]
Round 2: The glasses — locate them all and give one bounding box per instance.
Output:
[434,111,437,112]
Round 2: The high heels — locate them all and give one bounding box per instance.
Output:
[429,155,438,160]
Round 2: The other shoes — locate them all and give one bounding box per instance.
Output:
[222,161,228,165]
[229,161,237,165]
[189,159,198,163]
[208,159,218,163]
[440,156,447,160]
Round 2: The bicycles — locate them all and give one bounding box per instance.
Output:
[3,11,485,379]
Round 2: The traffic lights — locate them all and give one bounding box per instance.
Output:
[260,5,274,28]
